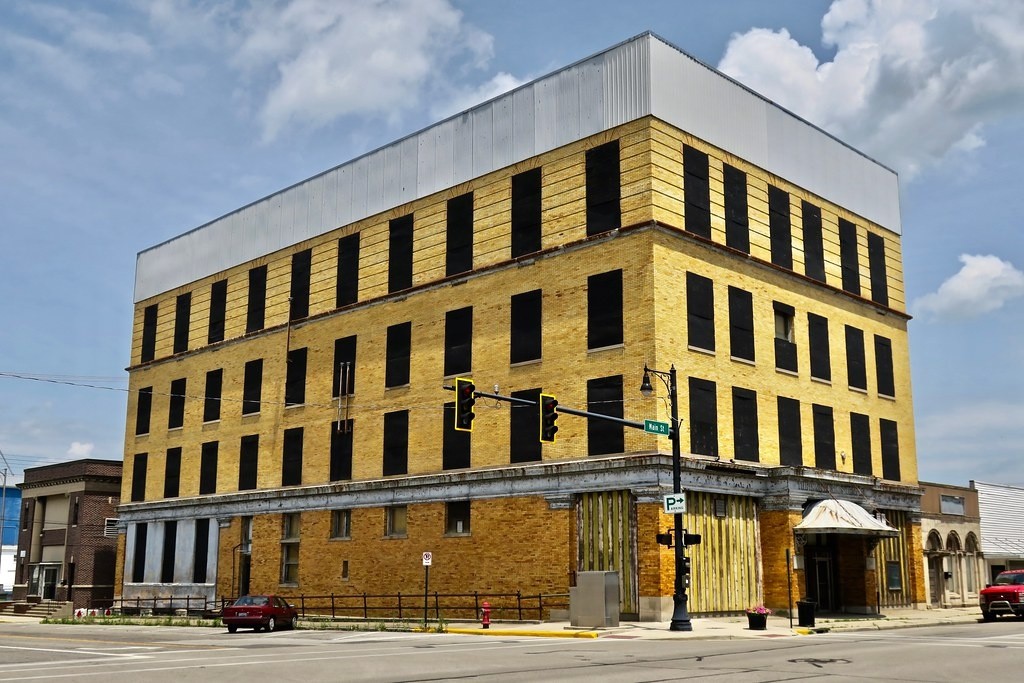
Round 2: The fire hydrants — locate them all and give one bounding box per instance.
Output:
[480,601,491,629]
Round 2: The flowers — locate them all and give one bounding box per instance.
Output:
[745,606,772,615]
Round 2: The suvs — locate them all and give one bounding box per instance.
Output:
[979,570,1024,622]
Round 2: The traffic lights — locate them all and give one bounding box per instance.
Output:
[539,393,559,444]
[454,377,476,433]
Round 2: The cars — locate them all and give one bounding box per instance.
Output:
[222,595,298,633]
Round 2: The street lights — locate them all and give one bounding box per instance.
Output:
[639,361,694,632]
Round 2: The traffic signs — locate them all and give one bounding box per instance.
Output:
[644,419,669,436]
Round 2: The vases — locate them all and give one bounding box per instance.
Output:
[746,614,768,630]
